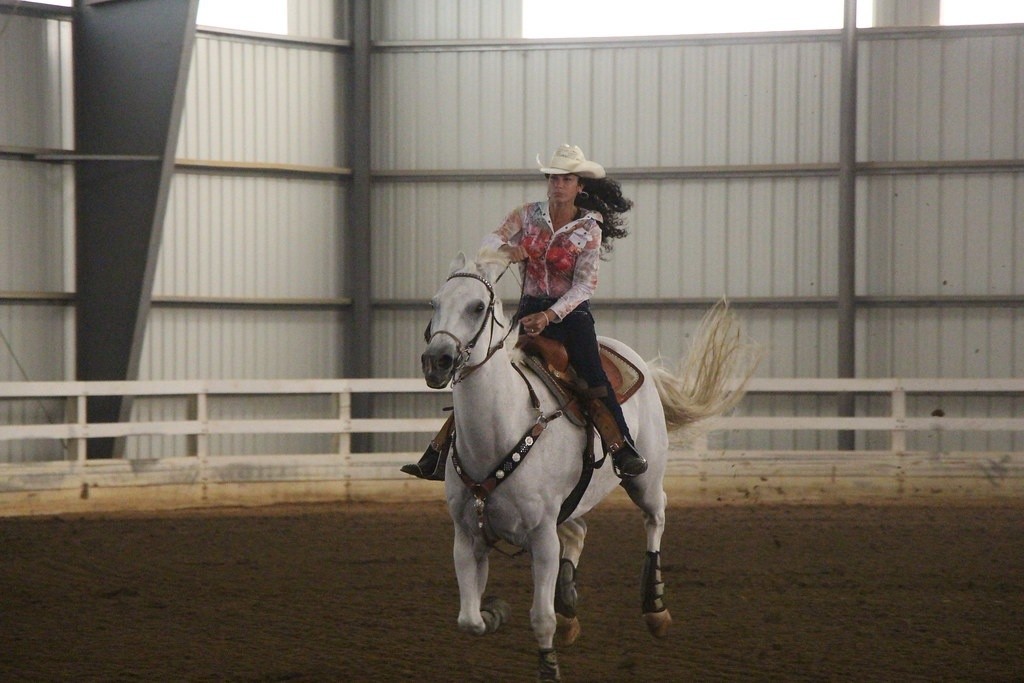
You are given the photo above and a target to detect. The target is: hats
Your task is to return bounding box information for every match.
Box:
[536,143,607,180]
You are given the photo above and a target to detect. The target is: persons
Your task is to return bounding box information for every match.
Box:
[400,144,648,481]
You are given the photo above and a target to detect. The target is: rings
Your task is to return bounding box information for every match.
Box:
[531,328,535,333]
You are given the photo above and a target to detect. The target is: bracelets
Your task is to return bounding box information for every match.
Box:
[540,312,549,326]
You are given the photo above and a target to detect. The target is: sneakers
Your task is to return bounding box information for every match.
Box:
[399,443,445,482]
[612,435,648,476]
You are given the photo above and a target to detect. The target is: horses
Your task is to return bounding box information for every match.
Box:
[420,250,764,682]
